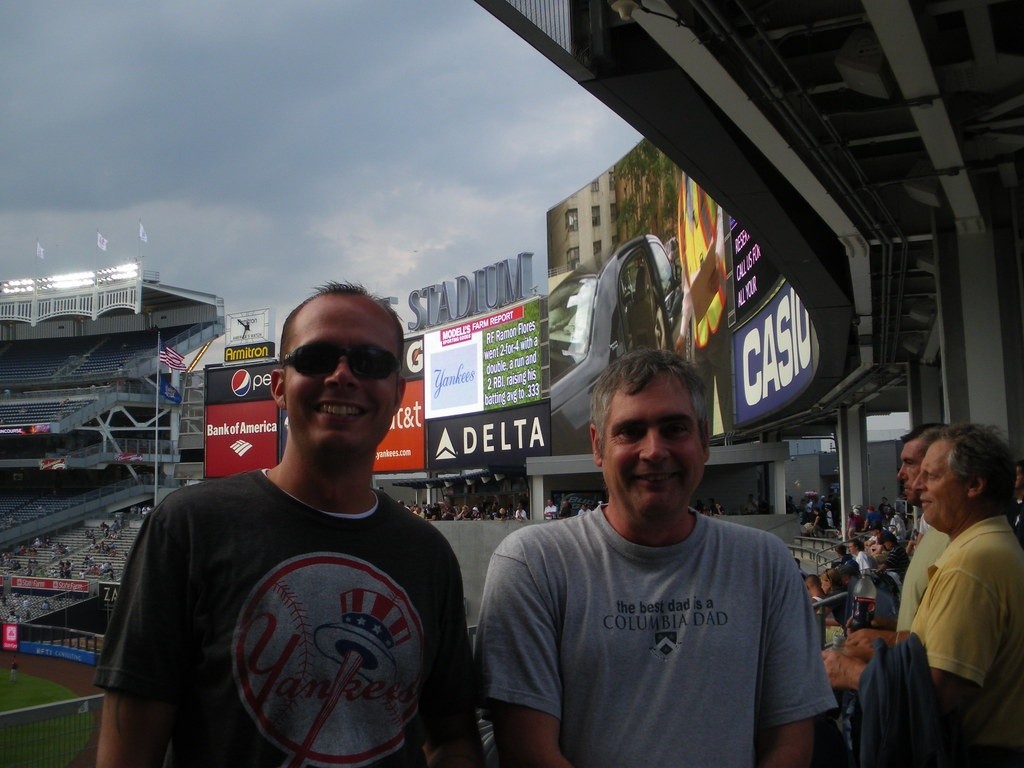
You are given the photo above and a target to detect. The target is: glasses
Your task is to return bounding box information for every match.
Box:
[281,341,399,381]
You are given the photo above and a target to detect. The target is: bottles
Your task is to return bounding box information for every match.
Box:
[850,569,878,630]
[823,633,851,719]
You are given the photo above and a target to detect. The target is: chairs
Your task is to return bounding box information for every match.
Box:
[0,320,213,621]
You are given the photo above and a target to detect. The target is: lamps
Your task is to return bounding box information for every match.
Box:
[888,364,902,374]
[444,481,455,488]
[481,477,492,484]
[915,241,935,274]
[465,479,476,486]
[902,158,949,209]
[902,335,927,354]
[835,28,896,101]
[425,484,434,489]
[910,300,931,324]
[494,473,506,481]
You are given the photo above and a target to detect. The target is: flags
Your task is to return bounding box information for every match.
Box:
[158,381,182,404]
[159,339,187,371]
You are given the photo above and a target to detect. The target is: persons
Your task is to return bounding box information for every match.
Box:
[0,587,52,624]
[95,283,475,768]
[475,350,841,768]
[11,660,17,681]
[792,423,1024,768]
[3,504,152,579]
[396,497,592,524]
